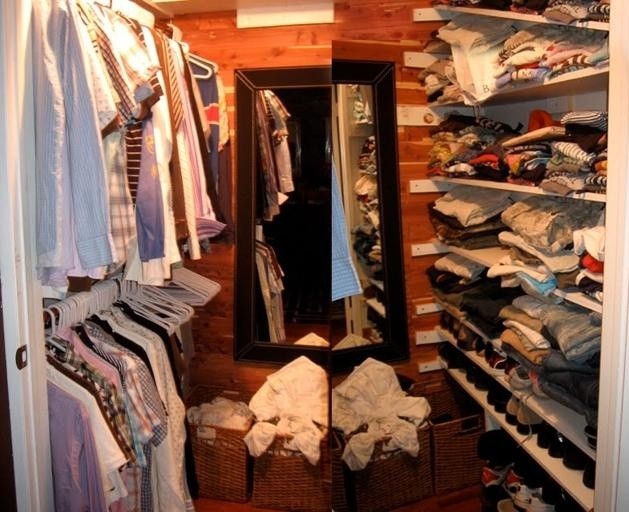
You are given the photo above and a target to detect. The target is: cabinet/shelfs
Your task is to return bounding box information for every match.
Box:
[436,0,628,512]
[339,86,388,343]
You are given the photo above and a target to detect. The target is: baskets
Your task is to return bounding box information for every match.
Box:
[188,379,483,512]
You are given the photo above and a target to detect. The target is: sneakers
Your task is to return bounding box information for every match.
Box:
[482,463,555,512]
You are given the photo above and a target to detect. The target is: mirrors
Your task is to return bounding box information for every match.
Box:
[333,57,409,366]
[233,67,336,364]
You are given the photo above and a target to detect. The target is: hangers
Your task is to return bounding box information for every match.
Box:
[42,265,222,405]
[28,0,222,78]
